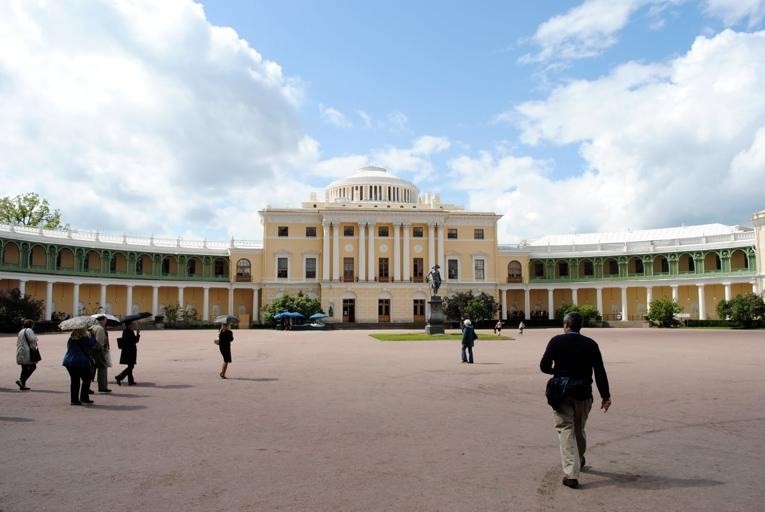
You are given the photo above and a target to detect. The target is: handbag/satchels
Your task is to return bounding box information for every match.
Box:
[30,346,41,362]
[88,350,97,368]
[103,350,114,368]
[545,375,572,411]
[473,331,478,341]
[116,337,125,349]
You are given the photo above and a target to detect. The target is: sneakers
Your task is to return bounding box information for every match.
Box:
[71,400,81,405]
[115,375,122,386]
[88,388,94,394]
[20,387,31,390]
[79,399,95,405]
[128,381,137,386]
[562,476,578,489]
[98,388,112,393]
[16,381,23,388]
[579,456,586,472]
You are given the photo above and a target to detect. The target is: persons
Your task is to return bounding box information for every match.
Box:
[494,318,507,335]
[15,319,40,390]
[51,307,141,406]
[212,322,234,378]
[459,318,479,364]
[540,311,613,489]
[428,264,442,295]
[520,321,527,335]
[273,316,320,330]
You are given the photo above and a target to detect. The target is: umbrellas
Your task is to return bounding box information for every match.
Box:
[273,312,327,319]
